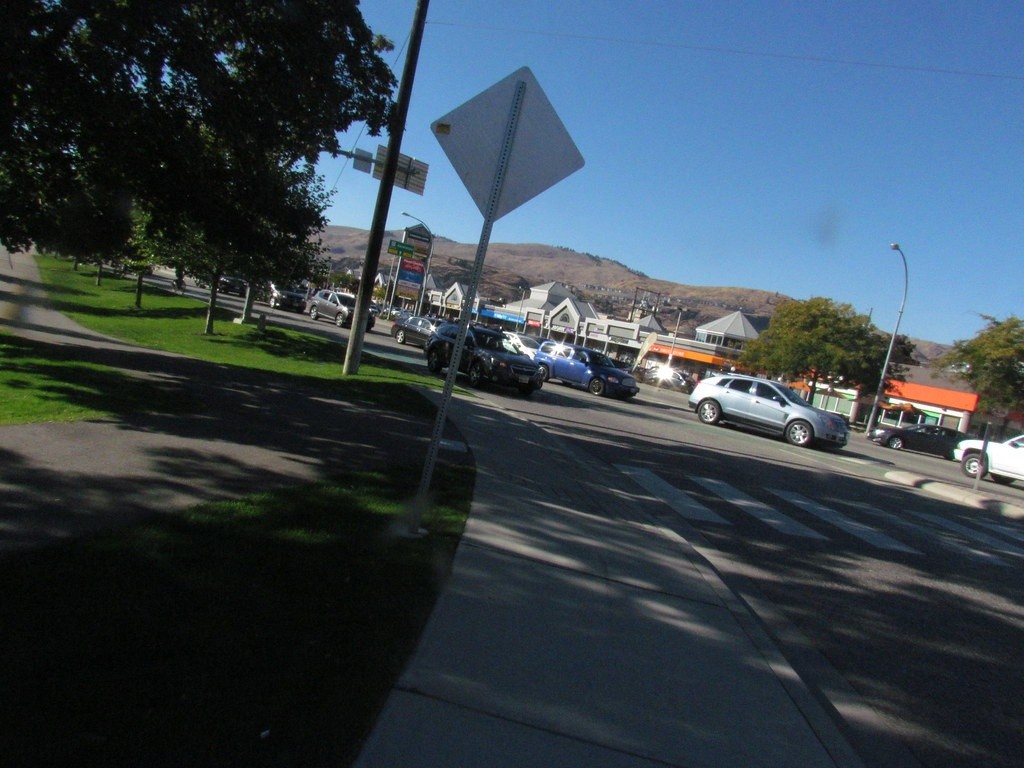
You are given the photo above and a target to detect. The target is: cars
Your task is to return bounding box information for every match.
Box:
[954,435,1024,483]
[867,423,970,461]
[487,329,540,360]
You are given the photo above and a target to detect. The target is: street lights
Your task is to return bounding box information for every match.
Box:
[515,286,525,328]
[666,307,684,368]
[864,244,910,436]
[402,212,435,316]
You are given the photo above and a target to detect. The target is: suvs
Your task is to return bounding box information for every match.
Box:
[424,317,541,397]
[534,341,642,399]
[687,373,850,449]
[192,267,447,351]
[644,365,694,392]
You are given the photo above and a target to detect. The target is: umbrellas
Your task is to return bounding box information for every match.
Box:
[784,381,930,438]
